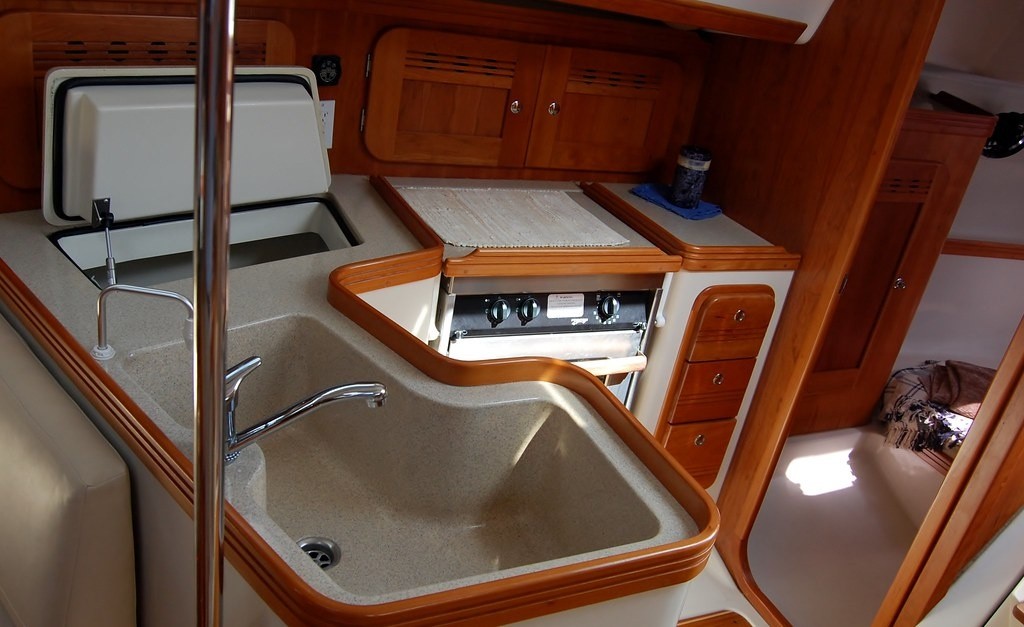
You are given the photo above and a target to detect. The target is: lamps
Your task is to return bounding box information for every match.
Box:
[911,77,1024,159]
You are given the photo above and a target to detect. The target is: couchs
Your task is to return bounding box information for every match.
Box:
[876,357,997,477]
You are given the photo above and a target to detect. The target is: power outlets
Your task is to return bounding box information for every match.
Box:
[320,99,336,149]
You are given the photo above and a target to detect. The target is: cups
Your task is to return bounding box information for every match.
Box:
[668,143,712,210]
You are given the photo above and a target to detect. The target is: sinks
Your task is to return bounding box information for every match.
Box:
[120,315,723,627]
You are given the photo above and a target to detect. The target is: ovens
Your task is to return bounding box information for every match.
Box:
[446,293,648,411]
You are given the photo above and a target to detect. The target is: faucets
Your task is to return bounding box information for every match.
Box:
[224,358,388,468]
[90,283,193,362]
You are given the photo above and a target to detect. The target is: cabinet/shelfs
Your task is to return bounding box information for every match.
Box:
[785,84,999,437]
[579,177,804,512]
